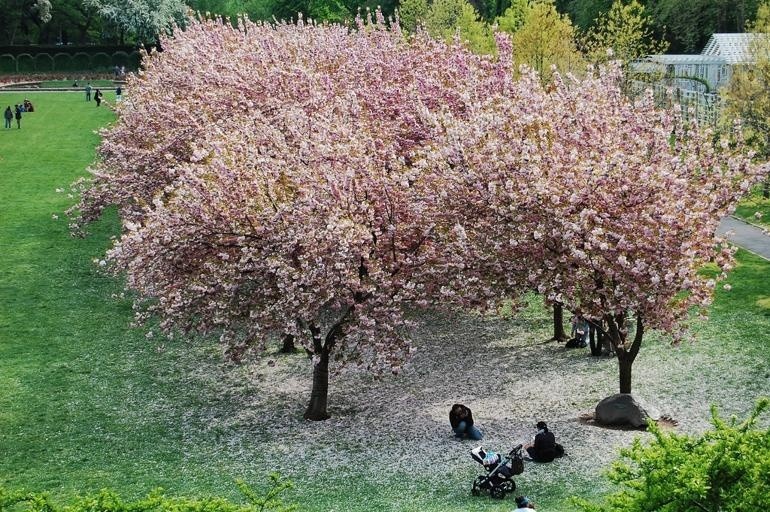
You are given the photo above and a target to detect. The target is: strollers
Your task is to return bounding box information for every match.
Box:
[469,442,526,501]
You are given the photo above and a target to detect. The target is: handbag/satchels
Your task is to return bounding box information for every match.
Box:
[566,338,587,349]
[512,450,524,475]
[553,442,564,458]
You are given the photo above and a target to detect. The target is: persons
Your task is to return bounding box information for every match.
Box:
[447,403,483,441]
[115,86,122,102]
[18,99,34,113]
[93,89,103,107]
[84,83,92,102]
[511,495,537,512]
[14,104,22,129]
[569,318,589,349]
[522,420,556,464]
[3,106,14,129]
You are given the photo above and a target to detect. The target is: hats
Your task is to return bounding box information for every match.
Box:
[516,496,530,505]
[536,421,547,429]
[575,307,586,315]
